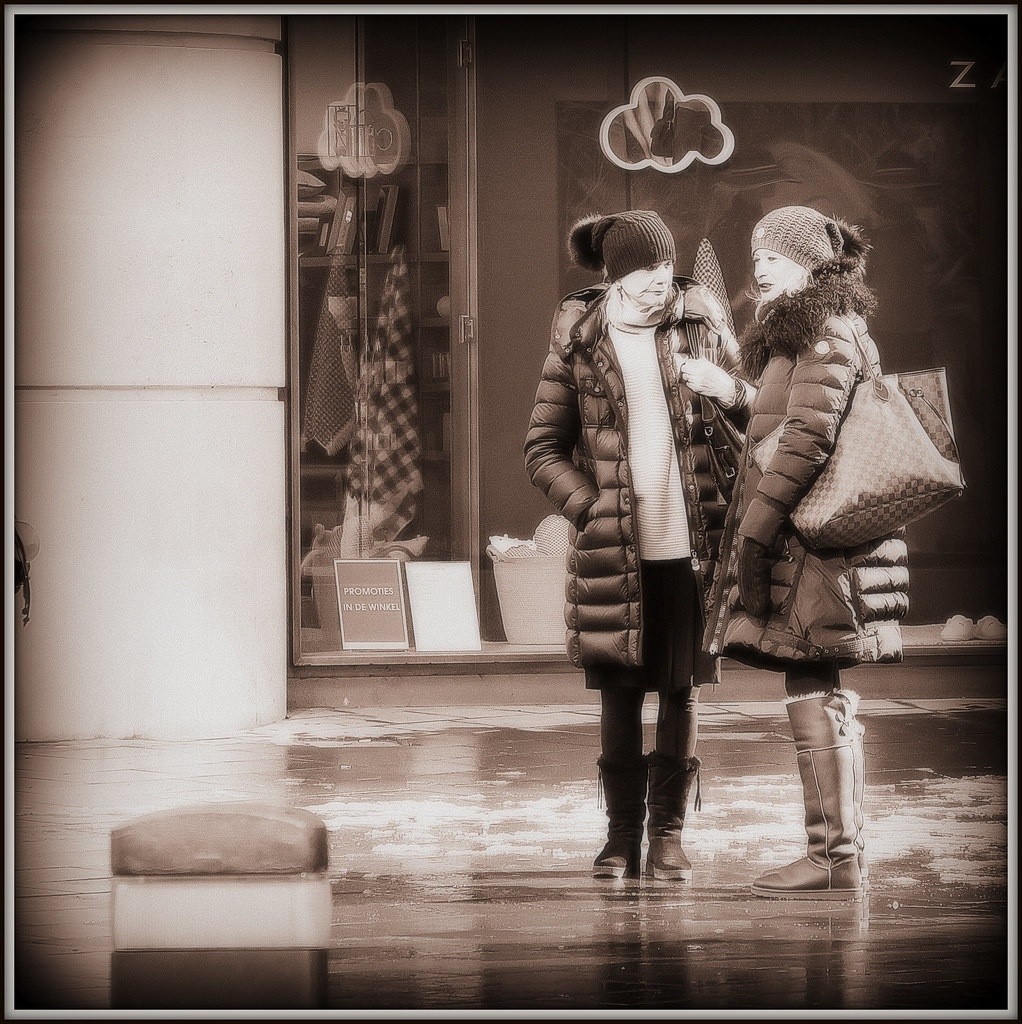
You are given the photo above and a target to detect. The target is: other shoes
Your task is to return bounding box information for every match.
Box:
[973,615,1006,639]
[942,616,974,641]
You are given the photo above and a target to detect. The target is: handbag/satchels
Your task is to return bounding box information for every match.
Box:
[684,318,747,504]
[748,314,965,548]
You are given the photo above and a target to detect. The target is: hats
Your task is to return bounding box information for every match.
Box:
[568,210,678,284]
[750,205,867,276]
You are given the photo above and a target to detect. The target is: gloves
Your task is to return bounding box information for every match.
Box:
[737,536,773,619]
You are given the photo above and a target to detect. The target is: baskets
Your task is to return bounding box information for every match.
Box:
[486,544,570,645]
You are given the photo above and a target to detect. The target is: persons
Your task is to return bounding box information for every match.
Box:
[701,204,909,900]
[523,210,757,880]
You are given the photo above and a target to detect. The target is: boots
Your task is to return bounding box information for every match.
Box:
[751,689,871,900]
[644,750,703,880]
[593,753,648,879]
